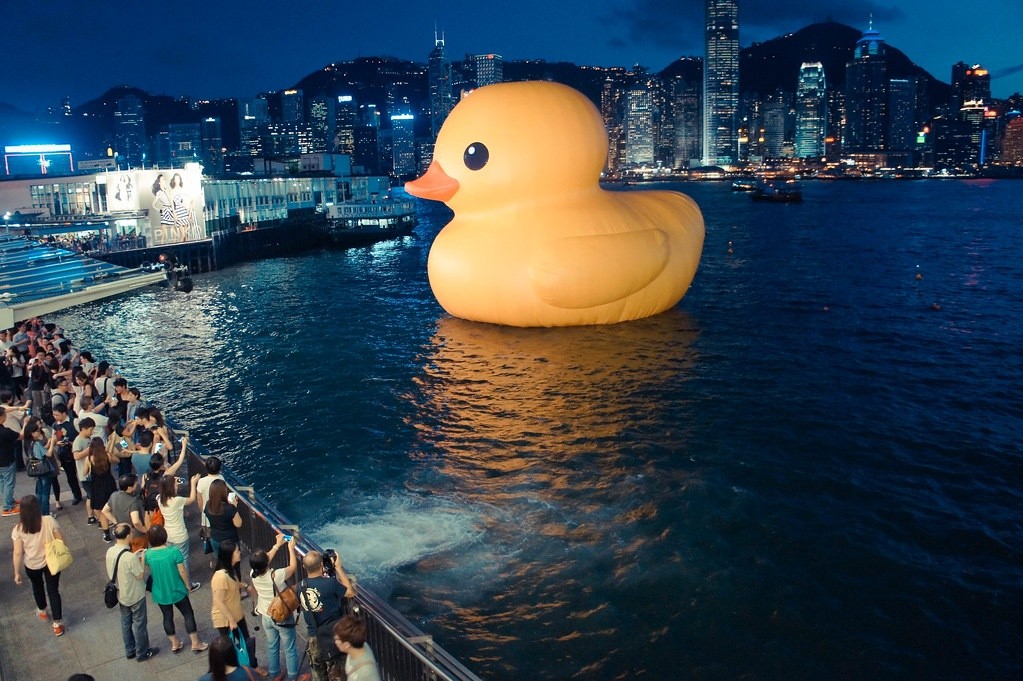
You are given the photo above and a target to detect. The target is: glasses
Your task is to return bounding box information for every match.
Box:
[333,637,342,641]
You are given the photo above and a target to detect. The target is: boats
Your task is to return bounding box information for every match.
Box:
[732,177,805,202]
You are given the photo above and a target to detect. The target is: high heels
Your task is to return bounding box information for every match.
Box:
[191,642,209,654]
[172,642,183,654]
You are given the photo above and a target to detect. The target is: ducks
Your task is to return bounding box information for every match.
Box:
[405,80,705,327]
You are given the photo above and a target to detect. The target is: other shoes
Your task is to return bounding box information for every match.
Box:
[2,507,21,516]
[138,647,160,662]
[13,498,21,506]
[255,663,311,681]
[188,582,200,592]
[53,625,64,636]
[127,653,136,660]
[16,465,27,472]
[14,400,22,406]
[37,608,48,623]
[47,497,116,543]
[239,589,248,600]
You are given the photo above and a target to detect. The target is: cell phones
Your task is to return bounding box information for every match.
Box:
[284,536,292,541]
[177,478,186,484]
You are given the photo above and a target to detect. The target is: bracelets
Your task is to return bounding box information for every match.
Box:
[273,546,278,550]
[103,401,107,404]
[17,406,18,411]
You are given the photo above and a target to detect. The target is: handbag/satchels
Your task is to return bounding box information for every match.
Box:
[203,539,214,555]
[104,428,136,457]
[228,626,259,669]
[151,507,164,526]
[44,516,73,576]
[94,395,109,417]
[26,441,52,477]
[266,570,300,623]
[103,580,119,608]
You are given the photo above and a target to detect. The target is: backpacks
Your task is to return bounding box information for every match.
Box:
[139,469,166,511]
[39,393,65,427]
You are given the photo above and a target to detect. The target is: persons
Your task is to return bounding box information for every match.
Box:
[41,234,127,251]
[0,317,381,681]
[152,173,200,244]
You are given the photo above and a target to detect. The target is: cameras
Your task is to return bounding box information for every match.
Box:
[62,439,72,449]
[321,549,336,568]
